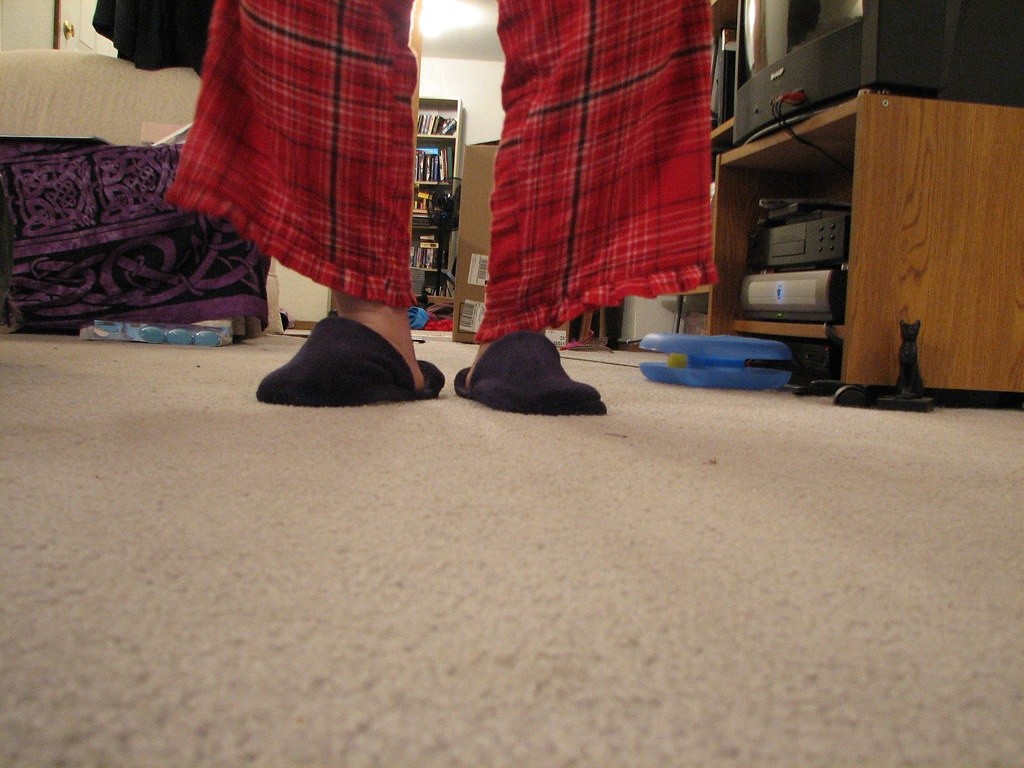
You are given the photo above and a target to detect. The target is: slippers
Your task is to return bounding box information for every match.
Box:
[256,317,447,407]
[453,331,609,416]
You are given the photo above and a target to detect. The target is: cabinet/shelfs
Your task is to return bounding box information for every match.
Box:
[707,94,1024,391]
[411,98,465,305]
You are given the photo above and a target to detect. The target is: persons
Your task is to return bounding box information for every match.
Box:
[168,0,718,416]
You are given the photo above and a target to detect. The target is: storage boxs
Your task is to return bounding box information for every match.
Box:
[453,141,569,347]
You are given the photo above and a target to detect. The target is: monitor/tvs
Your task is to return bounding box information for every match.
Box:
[732,0,943,147]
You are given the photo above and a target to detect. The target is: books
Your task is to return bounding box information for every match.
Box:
[411,234,446,270]
[415,147,453,182]
[413,190,441,226]
[426,287,445,296]
[418,114,457,134]
